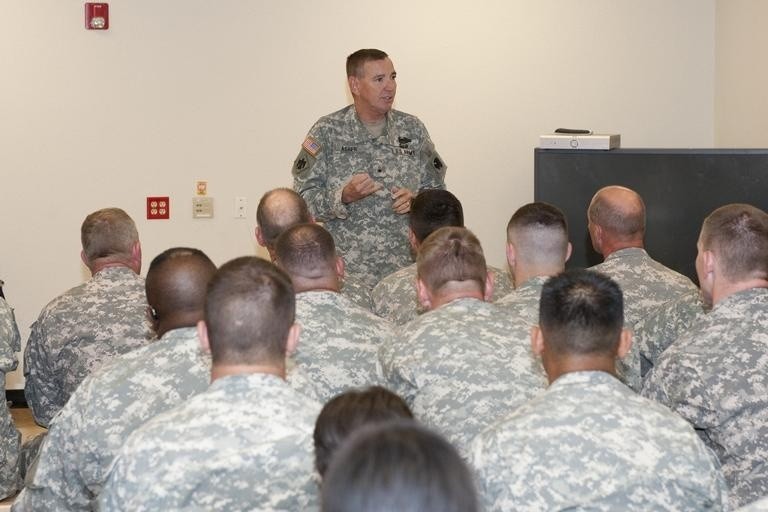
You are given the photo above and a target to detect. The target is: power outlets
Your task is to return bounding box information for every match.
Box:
[192,198,213,219]
[147,196,170,219]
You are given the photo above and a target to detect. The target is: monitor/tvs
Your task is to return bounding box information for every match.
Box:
[533,149,768,294]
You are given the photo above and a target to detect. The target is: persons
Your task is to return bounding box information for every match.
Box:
[291,48,447,309]
[0,185,768,512]
